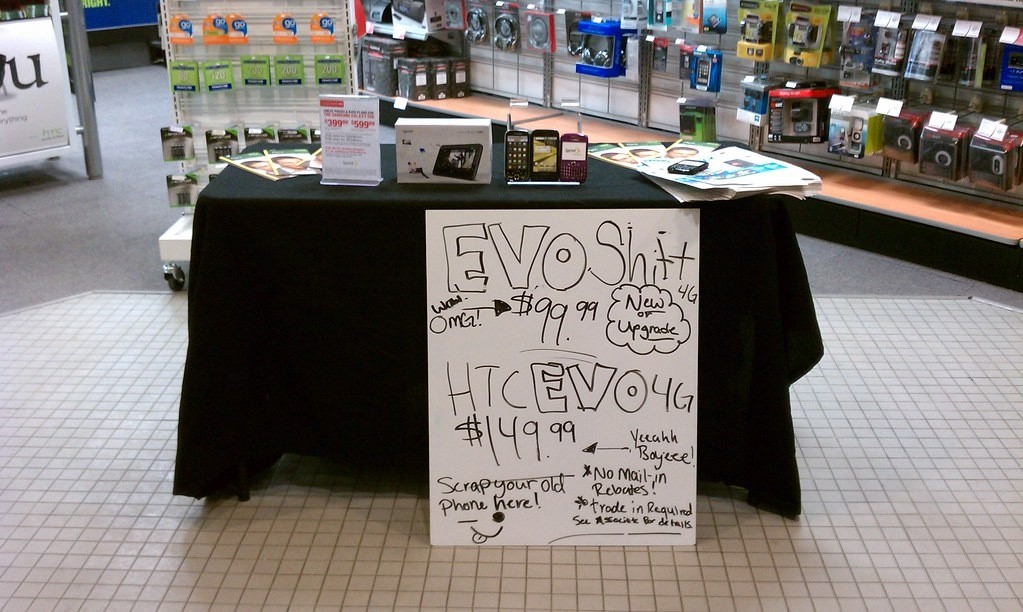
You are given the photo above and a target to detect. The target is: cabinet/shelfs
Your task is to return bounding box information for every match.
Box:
[0,0,104,182]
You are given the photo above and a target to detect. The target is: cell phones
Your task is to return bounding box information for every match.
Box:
[558,133,589,183]
[530,129,559,183]
[503,129,531,183]
[668,159,709,175]
[695,57,712,92]
[432,143,484,180]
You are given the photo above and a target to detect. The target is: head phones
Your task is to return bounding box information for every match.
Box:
[566,19,613,67]
[494,14,520,53]
[464,7,488,44]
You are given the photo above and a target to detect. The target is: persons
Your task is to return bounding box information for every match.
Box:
[630,148,661,159]
[272,155,322,175]
[241,159,276,176]
[601,153,638,165]
[310,152,323,168]
[664,146,698,158]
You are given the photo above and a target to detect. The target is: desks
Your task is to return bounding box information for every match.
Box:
[172,142,824,520]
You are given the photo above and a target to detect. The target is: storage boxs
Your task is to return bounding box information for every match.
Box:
[362,36,412,96]
[576,21,620,78]
[398,57,431,101]
[735,80,771,127]
[449,58,471,98]
[769,89,835,144]
[430,58,451,100]
[395,117,493,185]
[690,49,723,93]
